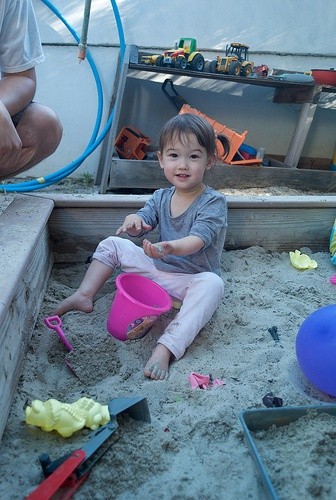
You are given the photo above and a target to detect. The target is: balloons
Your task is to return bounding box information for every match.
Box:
[295,304,336,396]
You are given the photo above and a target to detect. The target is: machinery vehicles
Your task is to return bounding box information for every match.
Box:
[157,38,206,72]
[211,41,253,77]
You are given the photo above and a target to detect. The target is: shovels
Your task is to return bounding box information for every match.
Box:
[44,315,97,381]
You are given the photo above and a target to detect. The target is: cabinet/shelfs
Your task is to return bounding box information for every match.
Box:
[91,43,335,196]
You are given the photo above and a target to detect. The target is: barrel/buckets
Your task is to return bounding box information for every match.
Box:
[106,273,173,342]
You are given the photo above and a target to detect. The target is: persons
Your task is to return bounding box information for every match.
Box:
[0,0,62,181]
[52,113,232,380]
